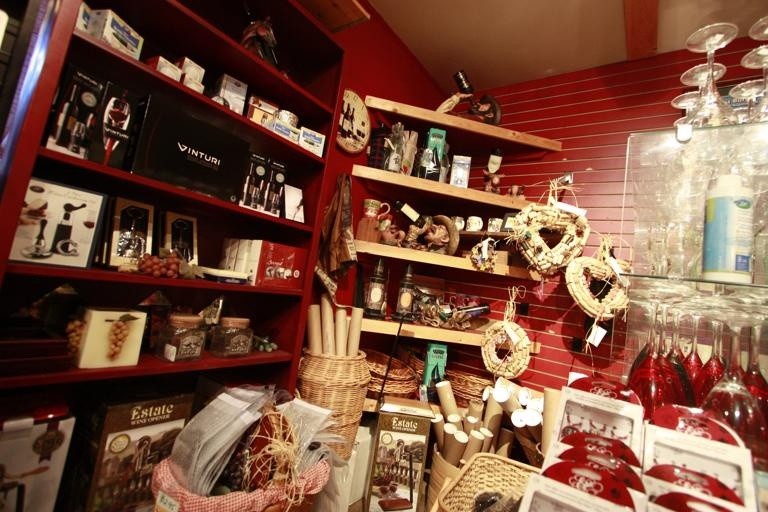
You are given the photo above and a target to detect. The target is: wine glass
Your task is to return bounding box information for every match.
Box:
[669,14,768,134]
[626,286,768,472]
[113,203,146,263]
[170,218,193,265]
[95,95,133,167]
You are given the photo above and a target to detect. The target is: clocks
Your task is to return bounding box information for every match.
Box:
[338,88,371,156]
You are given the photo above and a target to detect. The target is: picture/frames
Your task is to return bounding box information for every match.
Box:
[105,194,200,268]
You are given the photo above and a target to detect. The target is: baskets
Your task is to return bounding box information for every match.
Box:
[438,450,543,512]
[361,344,495,410]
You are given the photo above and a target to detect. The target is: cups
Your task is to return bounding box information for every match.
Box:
[356,118,367,142]
[450,214,466,231]
[67,120,87,153]
[486,217,504,232]
[360,197,391,220]
[465,215,484,232]
[250,188,260,210]
[270,193,280,215]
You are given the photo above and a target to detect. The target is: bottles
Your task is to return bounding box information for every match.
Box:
[242,162,256,206]
[384,122,405,173]
[389,260,420,324]
[392,198,435,233]
[54,82,80,149]
[359,255,392,322]
[209,316,256,359]
[699,171,759,285]
[263,169,275,212]
[49,201,91,256]
[158,312,211,362]
[334,98,355,139]
[412,286,492,331]
[453,68,479,109]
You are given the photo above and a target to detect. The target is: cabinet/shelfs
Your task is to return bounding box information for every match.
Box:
[337,93,561,427]
[0,0,347,493]
